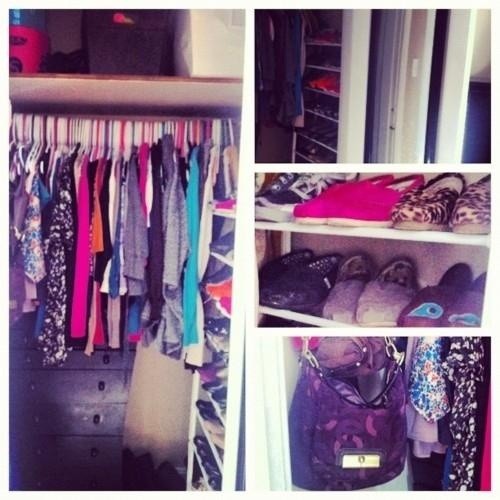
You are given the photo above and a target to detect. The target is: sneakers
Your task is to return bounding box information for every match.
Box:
[294,175,400,225]
[259,249,315,288]
[398,264,474,327]
[255,172,360,221]
[444,271,486,327]
[255,173,299,206]
[450,173,491,234]
[328,175,424,227]
[259,253,347,309]
[392,173,467,231]
[324,253,378,322]
[357,257,420,327]
[294,29,339,164]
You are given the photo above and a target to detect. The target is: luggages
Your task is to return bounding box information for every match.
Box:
[10,25,51,74]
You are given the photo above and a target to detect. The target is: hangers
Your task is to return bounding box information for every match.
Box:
[10,113,239,184]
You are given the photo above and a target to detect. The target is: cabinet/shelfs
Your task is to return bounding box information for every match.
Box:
[244,9,490,494]
[8,74,243,490]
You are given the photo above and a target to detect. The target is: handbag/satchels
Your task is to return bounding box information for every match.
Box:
[288,358,407,489]
